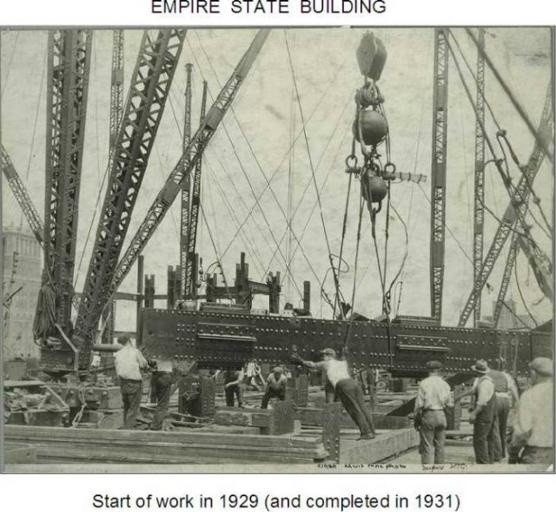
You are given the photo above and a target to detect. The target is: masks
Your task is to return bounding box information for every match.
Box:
[321,348,336,356]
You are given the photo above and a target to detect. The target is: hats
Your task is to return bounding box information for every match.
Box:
[471,359,490,374]
[528,357,553,377]
[426,360,442,370]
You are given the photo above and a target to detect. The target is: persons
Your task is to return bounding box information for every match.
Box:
[114,334,148,429]
[291,349,375,441]
[454,359,519,464]
[149,357,172,431]
[212,368,245,408]
[261,367,287,408]
[505,357,555,463]
[414,361,450,464]
